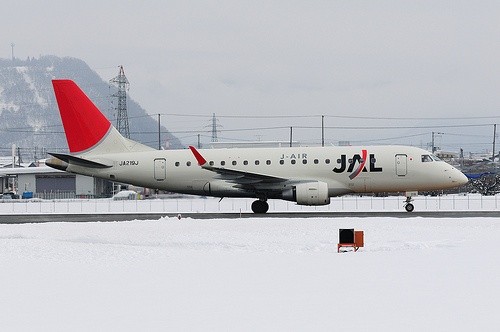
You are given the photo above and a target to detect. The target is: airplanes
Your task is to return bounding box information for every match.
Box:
[44,80,468,212]
